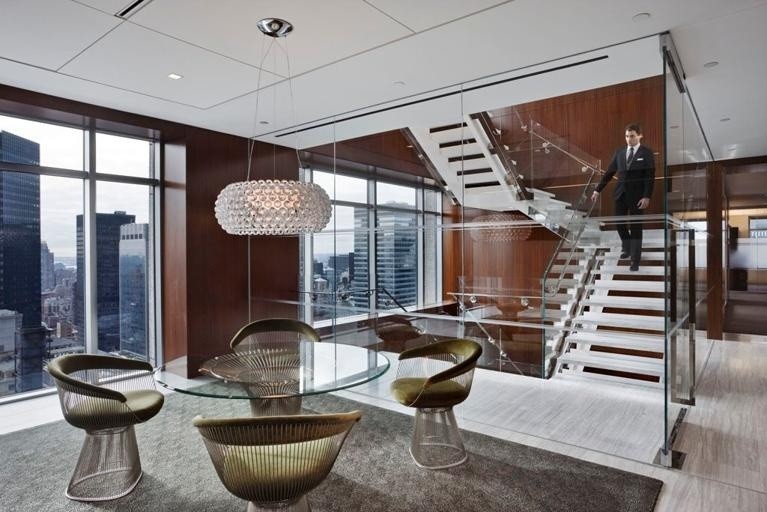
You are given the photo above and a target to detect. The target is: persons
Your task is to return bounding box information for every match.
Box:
[589,123,657,272]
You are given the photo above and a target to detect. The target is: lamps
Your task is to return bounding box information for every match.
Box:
[468,212,534,244]
[210,16,334,239]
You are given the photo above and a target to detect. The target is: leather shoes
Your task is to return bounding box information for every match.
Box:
[629,258,640,272]
[619,250,630,259]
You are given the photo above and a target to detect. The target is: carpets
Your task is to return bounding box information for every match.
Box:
[0,375,666,511]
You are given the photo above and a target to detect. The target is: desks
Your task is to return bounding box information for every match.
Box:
[152,338,392,417]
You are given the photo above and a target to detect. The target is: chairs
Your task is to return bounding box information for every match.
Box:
[41,351,169,502]
[228,315,322,417]
[186,408,369,511]
[388,337,485,470]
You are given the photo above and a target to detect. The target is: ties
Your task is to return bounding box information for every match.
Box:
[627,147,635,165]
